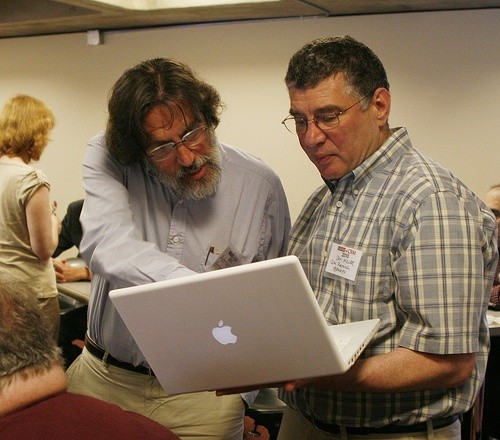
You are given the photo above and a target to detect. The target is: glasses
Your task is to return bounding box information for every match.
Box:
[141,111,210,162]
[281,86,385,135]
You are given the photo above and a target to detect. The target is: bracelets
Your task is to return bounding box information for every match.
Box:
[85,267,90,279]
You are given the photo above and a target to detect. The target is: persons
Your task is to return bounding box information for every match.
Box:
[0,94,60,347]
[64,57,292,440]
[0,268,182,440]
[276,34,500,440]
[51,199,92,282]
[480,185,500,310]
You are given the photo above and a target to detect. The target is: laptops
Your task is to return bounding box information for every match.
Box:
[108,255,381,394]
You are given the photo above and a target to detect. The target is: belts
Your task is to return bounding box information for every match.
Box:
[302,412,459,436]
[85,335,157,380]
